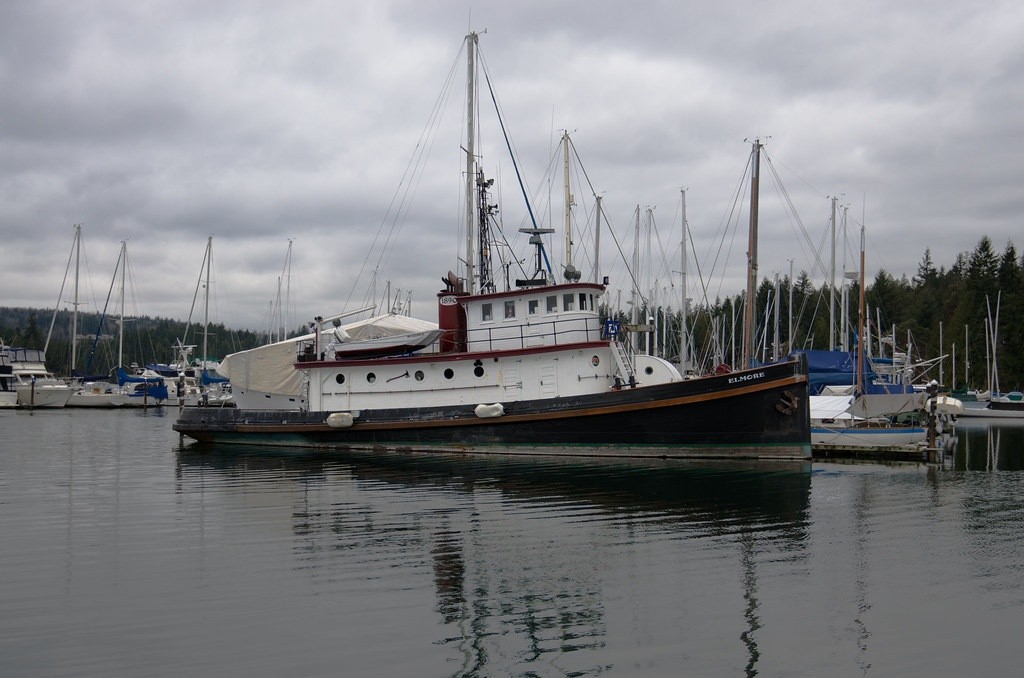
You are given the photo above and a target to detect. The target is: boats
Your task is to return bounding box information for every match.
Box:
[0,336,21,408]
[11,348,84,408]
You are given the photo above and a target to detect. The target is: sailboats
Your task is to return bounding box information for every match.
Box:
[43,222,132,407]
[580,194,1023,434]
[172,32,813,476]
[85,240,168,407]
[136,236,292,407]
